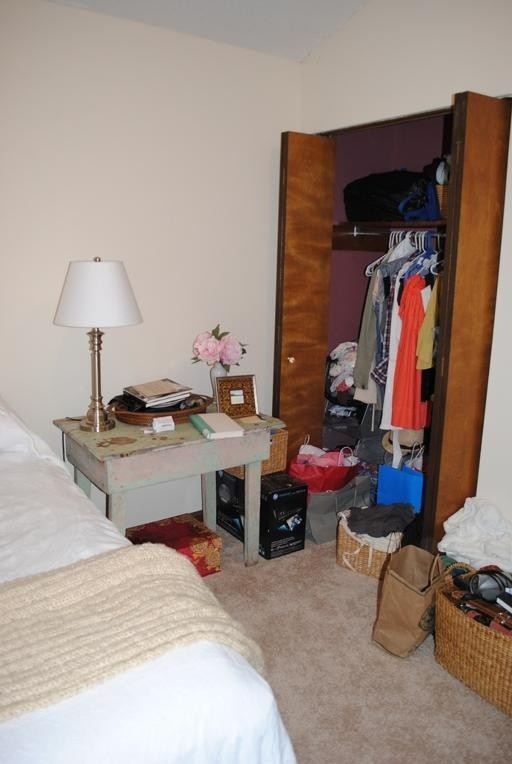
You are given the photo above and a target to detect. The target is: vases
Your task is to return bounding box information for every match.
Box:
[209,362,228,404]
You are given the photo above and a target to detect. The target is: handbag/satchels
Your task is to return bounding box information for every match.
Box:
[372,533,446,659]
[376,441,425,519]
[288,433,360,492]
[305,476,370,545]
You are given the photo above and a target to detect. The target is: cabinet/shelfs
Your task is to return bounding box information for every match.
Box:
[269,90,511,559]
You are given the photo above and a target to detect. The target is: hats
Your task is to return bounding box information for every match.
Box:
[382,429,424,455]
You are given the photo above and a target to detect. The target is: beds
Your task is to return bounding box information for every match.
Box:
[1,405,302,764]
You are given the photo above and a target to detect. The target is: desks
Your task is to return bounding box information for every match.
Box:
[51,413,275,568]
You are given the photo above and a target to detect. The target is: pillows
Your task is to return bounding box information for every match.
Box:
[1,402,54,454]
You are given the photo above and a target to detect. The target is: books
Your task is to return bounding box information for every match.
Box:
[496,592,512,613]
[125,378,193,404]
[189,412,244,439]
[123,391,191,409]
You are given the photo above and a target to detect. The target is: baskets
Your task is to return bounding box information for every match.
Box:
[106,394,213,427]
[223,429,289,479]
[434,563,512,717]
[336,524,391,581]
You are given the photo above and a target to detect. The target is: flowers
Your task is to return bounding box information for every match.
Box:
[190,323,253,373]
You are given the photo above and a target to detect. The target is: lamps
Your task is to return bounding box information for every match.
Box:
[51,257,145,433]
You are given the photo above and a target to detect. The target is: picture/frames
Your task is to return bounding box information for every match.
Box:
[213,374,257,420]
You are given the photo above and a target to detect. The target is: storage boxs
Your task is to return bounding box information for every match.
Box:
[125,514,221,580]
[215,469,309,561]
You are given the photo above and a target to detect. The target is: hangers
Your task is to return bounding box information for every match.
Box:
[416,232,443,276]
[399,230,405,242]
[366,231,393,274]
[409,232,429,270]
[418,232,422,253]
[430,260,444,276]
[410,232,427,264]
[414,232,419,249]
[405,232,438,278]
[364,231,396,278]
[381,231,401,264]
